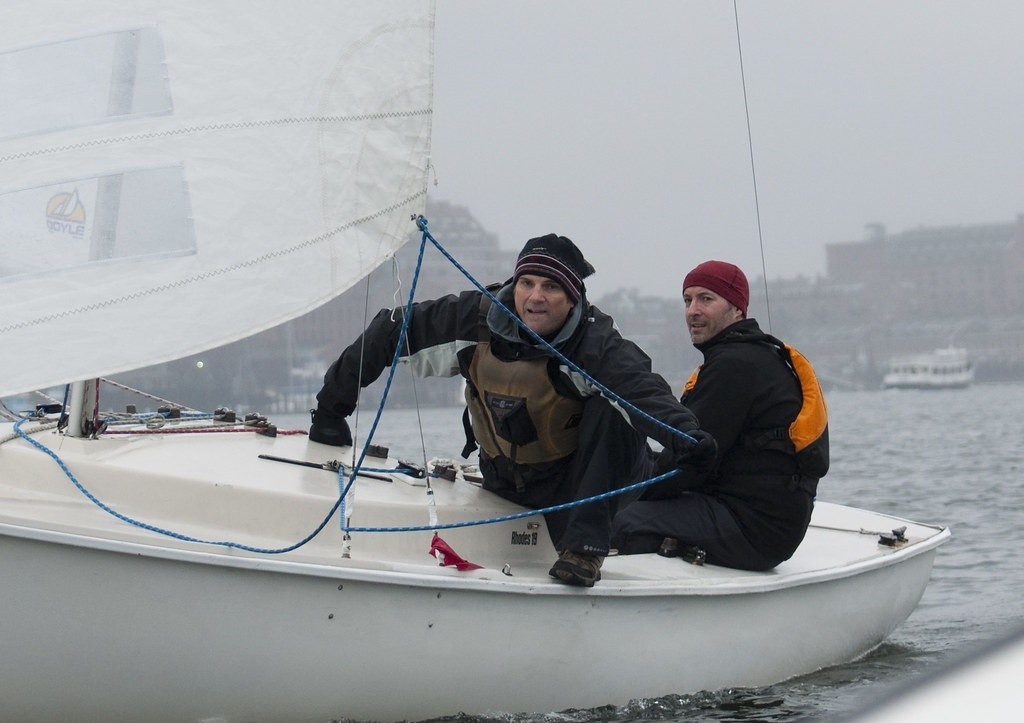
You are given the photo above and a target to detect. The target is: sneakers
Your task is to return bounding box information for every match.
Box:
[549,549,605,588]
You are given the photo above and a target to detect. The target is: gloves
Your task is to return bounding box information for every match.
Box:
[675,430,719,476]
[308,402,352,447]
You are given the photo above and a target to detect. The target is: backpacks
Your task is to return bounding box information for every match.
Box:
[750,332,829,477]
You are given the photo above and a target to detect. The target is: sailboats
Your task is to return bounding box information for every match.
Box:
[2,1,953,723]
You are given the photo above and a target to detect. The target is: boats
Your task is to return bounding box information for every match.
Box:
[882,343,977,389]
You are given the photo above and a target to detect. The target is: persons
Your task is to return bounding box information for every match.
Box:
[609,259,830,572]
[308,234,720,587]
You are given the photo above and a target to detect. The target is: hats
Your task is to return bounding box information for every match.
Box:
[683,259,750,319]
[514,232,586,306]
